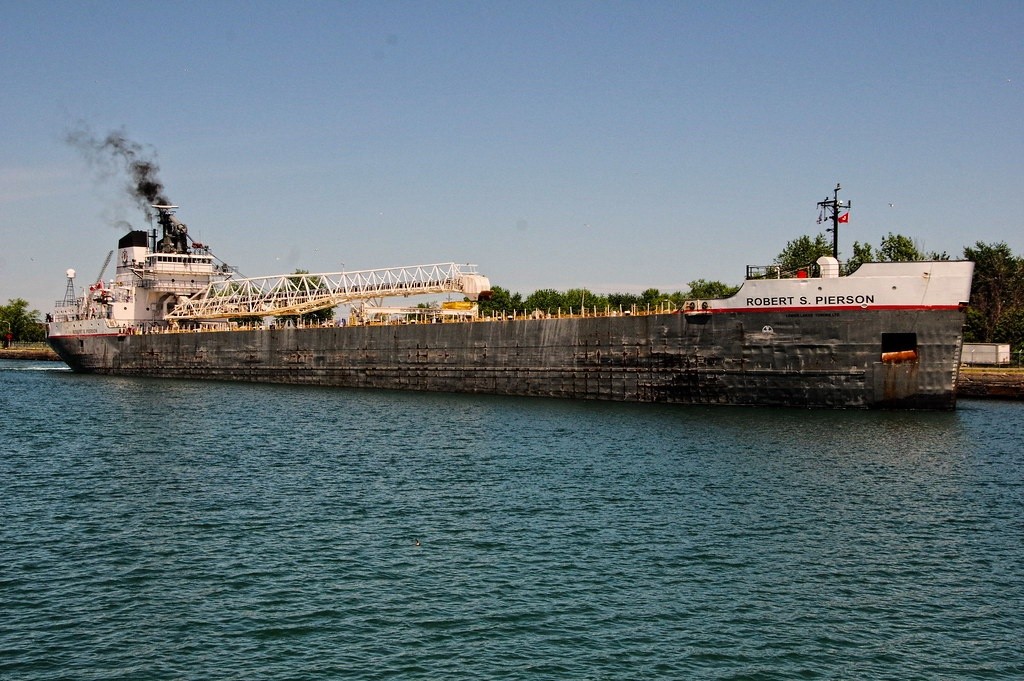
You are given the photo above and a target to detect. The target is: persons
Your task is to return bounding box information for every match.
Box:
[123,323,135,335]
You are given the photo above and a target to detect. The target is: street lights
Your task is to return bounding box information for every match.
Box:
[3,321,10,347]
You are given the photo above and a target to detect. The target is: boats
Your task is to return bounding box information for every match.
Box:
[45,180,975,411]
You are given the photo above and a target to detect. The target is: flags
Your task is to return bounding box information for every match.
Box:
[839,213,848,223]
